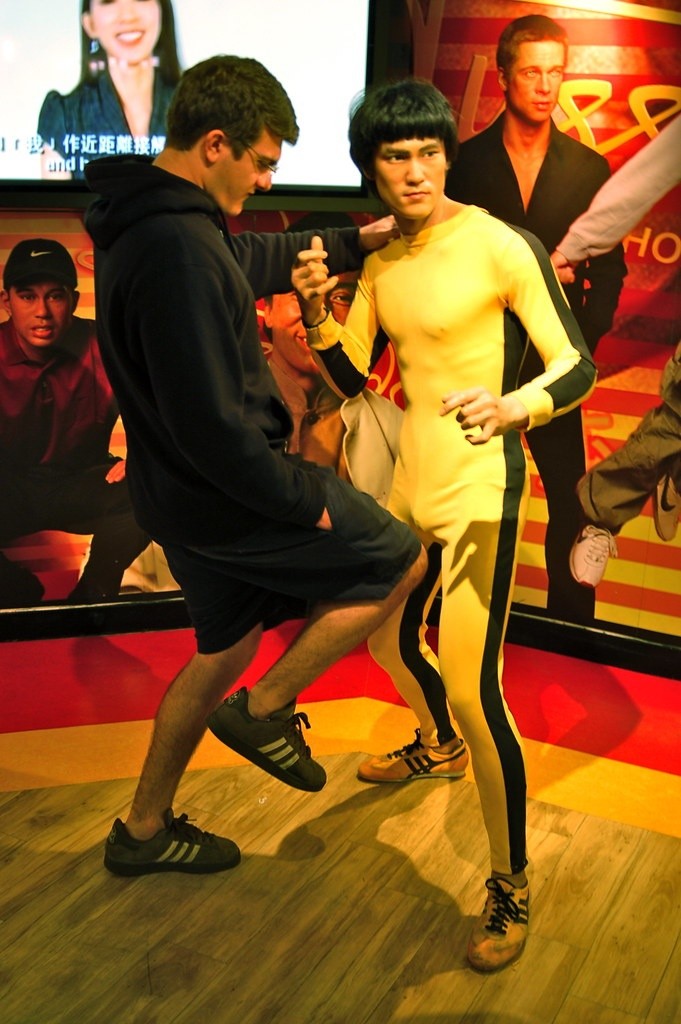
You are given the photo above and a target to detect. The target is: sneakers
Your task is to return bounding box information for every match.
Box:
[569,522,617,587]
[467,878,530,972]
[205,686,327,793]
[359,728,469,782]
[652,474,681,541]
[104,807,241,876]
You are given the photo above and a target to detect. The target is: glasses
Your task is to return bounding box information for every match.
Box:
[225,133,280,179]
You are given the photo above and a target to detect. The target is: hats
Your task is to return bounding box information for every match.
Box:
[3,239,78,290]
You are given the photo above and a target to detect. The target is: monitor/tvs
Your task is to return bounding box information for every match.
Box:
[0,0,388,213]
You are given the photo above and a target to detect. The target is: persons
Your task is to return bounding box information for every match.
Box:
[549,116,680,588]
[77,56,429,875]
[38,0,182,180]
[0,238,152,608]
[444,13,627,621]
[264,213,404,526]
[292,81,598,971]
